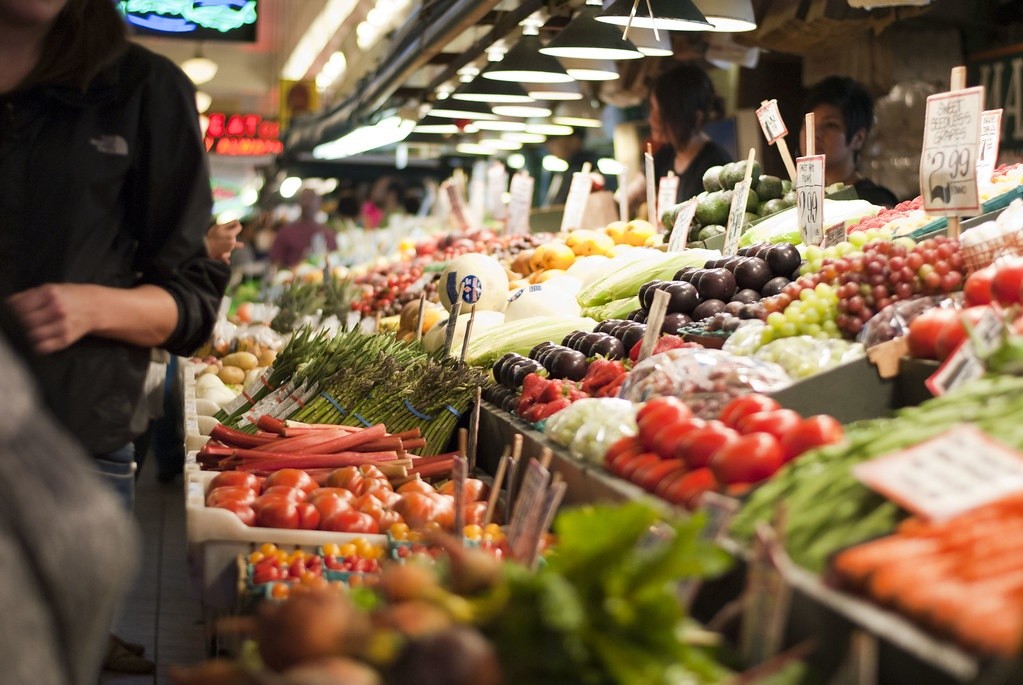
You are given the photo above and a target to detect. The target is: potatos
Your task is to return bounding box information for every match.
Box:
[194,351,276,384]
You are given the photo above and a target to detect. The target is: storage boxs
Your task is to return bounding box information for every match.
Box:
[176,183,896,591]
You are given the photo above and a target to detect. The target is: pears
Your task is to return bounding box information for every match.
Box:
[512,217,664,285]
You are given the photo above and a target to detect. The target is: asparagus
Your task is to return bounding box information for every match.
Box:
[206,323,489,456]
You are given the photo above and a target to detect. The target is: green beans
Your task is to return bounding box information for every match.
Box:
[730,330,1023,572]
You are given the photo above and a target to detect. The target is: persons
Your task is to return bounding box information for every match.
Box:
[796,75,900,210]
[95,217,246,677]
[612,61,738,208]
[269,175,409,273]
[545,122,603,206]
[0,0,232,523]
[0,340,145,684]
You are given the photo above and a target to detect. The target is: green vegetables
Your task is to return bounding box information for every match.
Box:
[268,259,363,333]
[461,490,833,685]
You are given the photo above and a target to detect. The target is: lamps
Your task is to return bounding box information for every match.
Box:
[491,97,552,118]
[554,83,603,127]
[452,61,534,103]
[481,35,574,83]
[538,10,645,60]
[594,0,715,31]
[558,57,622,80]
[529,82,579,100]
[412,115,459,133]
[620,25,674,57]
[427,89,497,120]
[692,0,757,32]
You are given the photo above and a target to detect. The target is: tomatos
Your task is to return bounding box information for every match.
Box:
[904,252,1023,367]
[204,463,490,536]
[248,521,556,599]
[602,392,840,507]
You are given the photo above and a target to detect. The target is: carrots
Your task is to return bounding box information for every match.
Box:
[830,486,1023,667]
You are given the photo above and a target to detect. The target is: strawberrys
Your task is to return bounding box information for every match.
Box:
[352,265,424,312]
[514,333,706,423]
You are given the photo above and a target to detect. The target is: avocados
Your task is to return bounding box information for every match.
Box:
[655,158,797,245]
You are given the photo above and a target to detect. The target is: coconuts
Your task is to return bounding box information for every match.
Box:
[421,253,581,354]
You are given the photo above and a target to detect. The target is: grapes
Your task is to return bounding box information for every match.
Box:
[751,225,964,348]
